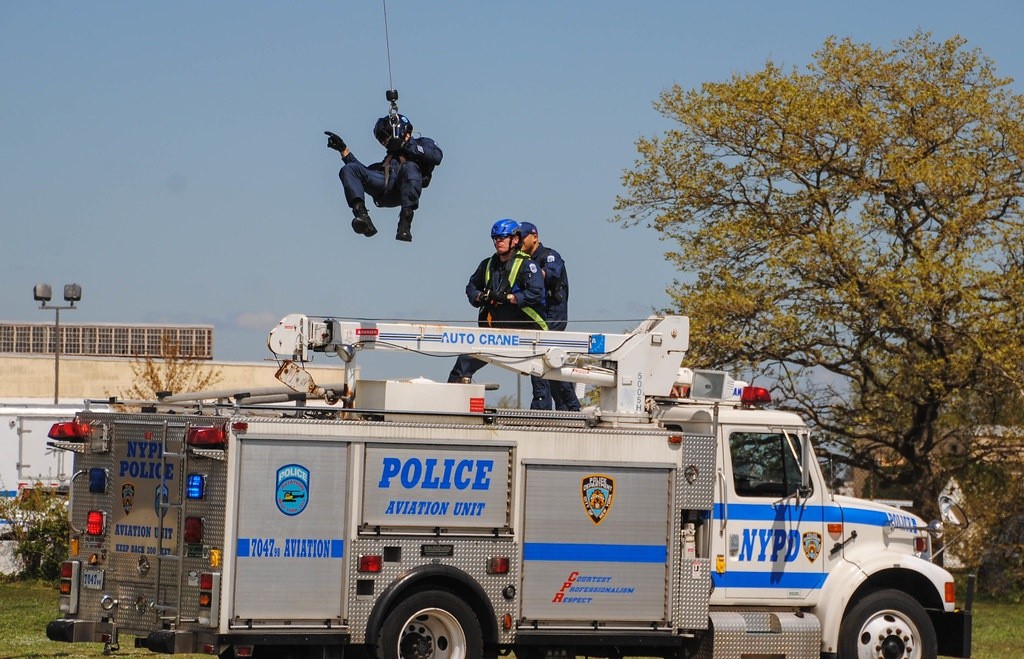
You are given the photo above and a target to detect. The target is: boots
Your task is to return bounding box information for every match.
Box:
[351,201,377,237]
[396,208,414,241]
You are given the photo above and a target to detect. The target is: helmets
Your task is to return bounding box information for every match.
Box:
[491,219,522,236]
[374,114,413,149]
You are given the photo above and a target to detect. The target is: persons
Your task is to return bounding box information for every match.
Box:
[324,113,443,242]
[448,219,582,412]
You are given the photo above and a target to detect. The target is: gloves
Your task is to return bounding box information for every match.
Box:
[324,132,346,152]
[386,137,404,153]
[480,287,511,310]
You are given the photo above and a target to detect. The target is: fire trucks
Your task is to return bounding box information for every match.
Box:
[42,315,981,659]
[0,398,121,501]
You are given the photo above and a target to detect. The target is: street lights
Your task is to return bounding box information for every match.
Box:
[32,282,84,405]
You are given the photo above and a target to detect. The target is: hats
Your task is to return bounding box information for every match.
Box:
[519,222,538,238]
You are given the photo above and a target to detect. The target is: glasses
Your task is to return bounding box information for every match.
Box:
[494,236,507,240]
